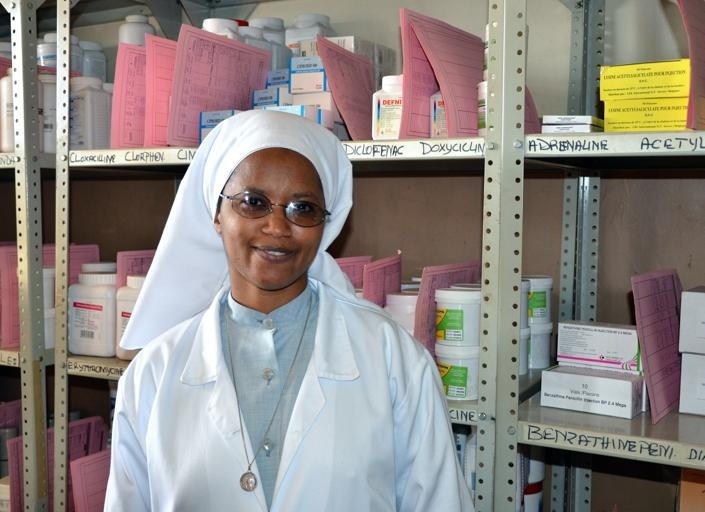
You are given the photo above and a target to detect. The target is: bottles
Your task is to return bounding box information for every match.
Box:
[201,10,340,69]
[117,13,156,48]
[0,427,19,478]
[369,72,446,141]
[522,445,547,512]
[381,275,422,338]
[0,33,115,155]
[41,260,147,362]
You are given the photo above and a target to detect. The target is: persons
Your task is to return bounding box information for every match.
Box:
[101,109,477,512]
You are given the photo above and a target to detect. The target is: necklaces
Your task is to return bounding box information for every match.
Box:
[224,283,313,491]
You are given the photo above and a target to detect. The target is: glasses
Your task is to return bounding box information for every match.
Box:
[219,192,330,227]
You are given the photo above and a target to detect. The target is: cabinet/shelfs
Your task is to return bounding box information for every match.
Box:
[490,0,705,512]
[0,0,182,512]
[53,0,587,512]
